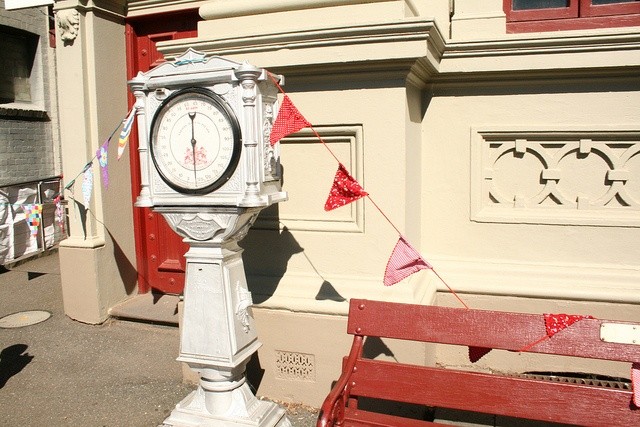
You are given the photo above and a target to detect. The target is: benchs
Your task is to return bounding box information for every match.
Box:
[315,298,640,427]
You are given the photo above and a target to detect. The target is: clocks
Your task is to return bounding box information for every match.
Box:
[147,86,244,197]
